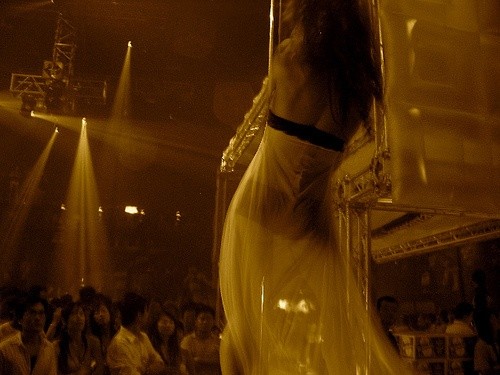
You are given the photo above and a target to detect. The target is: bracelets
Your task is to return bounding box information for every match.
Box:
[49,323,56,326]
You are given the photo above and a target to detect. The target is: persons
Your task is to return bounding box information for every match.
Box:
[219,0,385,375]
[0,284,500,375]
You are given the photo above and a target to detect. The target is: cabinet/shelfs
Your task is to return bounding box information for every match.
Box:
[392,334,478,375]
